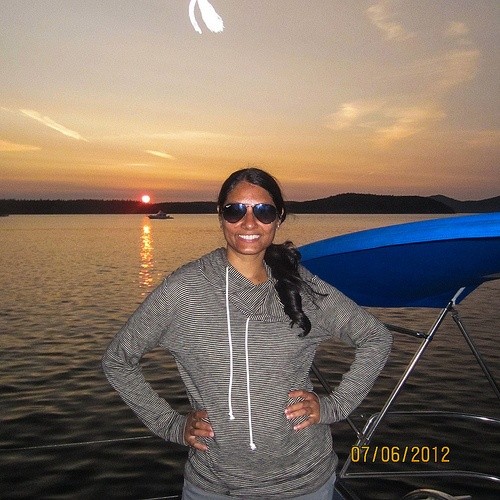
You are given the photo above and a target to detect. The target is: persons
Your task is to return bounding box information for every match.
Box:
[101,167,397,500]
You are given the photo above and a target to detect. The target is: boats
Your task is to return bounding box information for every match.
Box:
[148,210,174,219]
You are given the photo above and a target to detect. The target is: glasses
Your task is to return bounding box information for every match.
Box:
[219,203,281,225]
